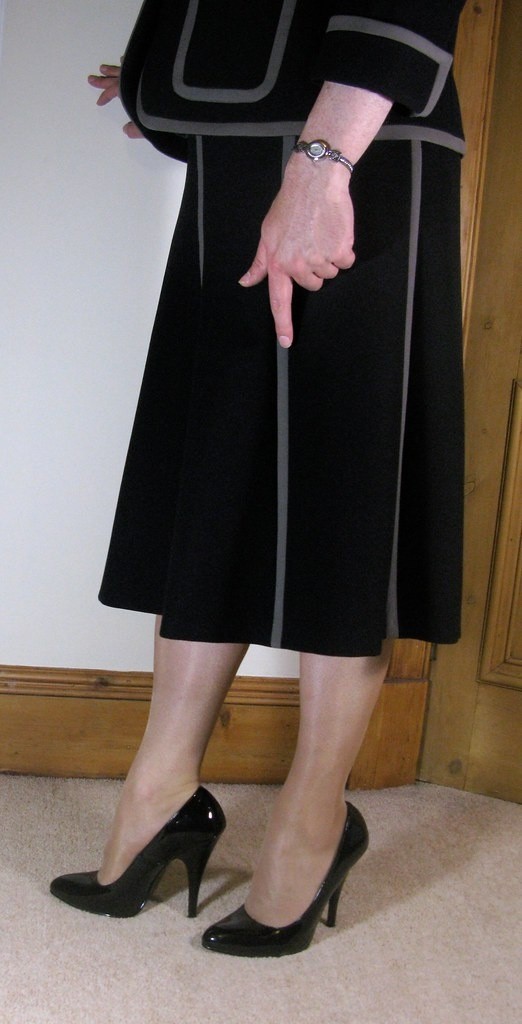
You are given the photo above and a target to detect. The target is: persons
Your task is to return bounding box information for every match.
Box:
[48,2,470,960]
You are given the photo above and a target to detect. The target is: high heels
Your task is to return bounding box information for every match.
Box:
[49,788,228,918]
[199,799,369,956]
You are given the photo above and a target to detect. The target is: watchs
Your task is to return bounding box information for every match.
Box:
[296,139,353,172]
